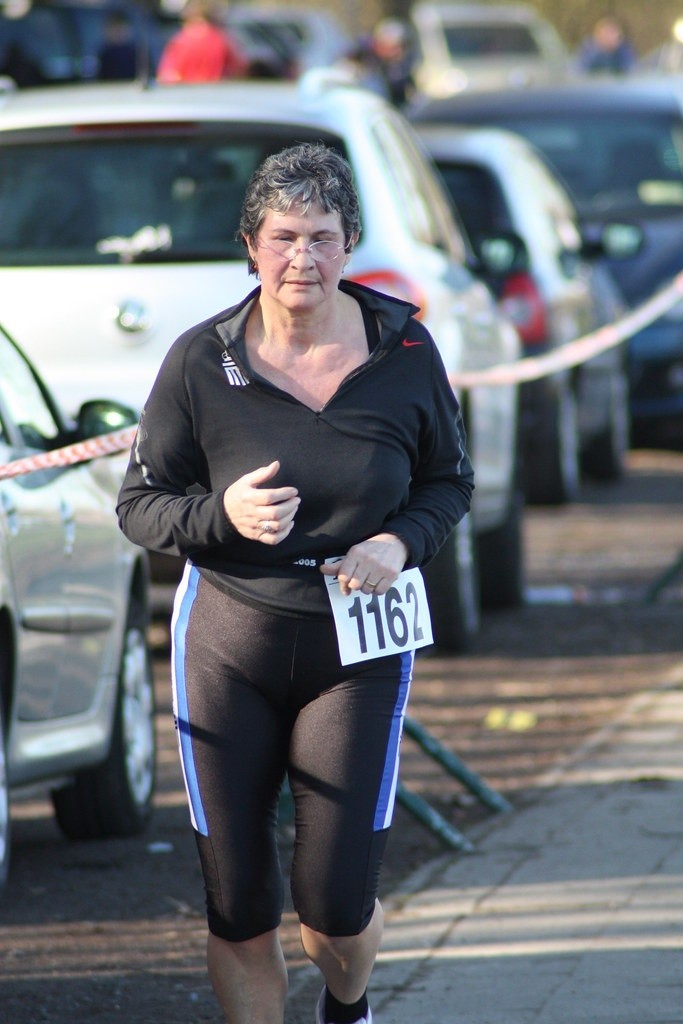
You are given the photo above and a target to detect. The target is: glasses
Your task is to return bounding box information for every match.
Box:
[261,231,354,262]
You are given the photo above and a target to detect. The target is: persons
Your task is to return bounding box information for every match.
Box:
[115,145,476,1024]
[156,0,249,82]
[96,16,141,80]
[344,23,413,104]
[579,20,637,75]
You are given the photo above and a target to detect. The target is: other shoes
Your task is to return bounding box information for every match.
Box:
[314,983,372,1024]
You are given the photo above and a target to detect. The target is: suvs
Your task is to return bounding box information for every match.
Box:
[0,64,534,662]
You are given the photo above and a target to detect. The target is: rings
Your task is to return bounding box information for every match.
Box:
[262,521,273,534]
[365,580,376,588]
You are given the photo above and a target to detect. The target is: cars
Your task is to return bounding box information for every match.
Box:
[0,332,157,852]
[107,2,683,505]
